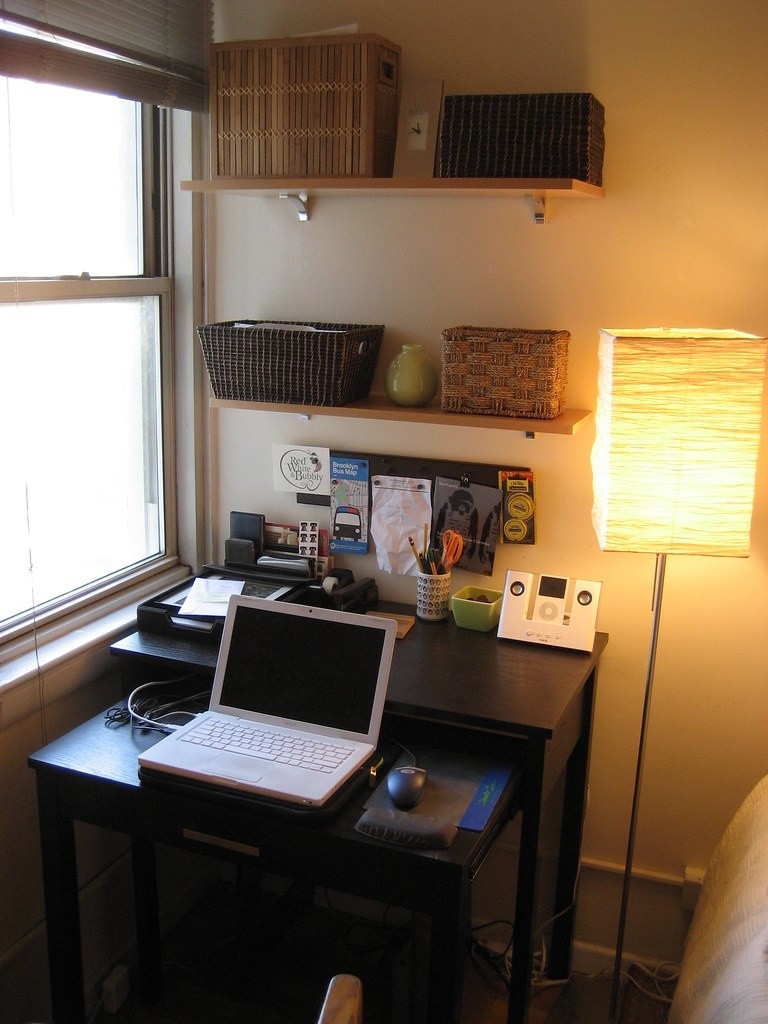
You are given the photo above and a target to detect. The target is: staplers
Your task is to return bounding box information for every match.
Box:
[333,577,378,614]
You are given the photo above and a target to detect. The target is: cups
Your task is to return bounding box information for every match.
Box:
[416,571,452,622]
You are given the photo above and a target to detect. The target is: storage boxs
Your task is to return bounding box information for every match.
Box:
[102,963,131,1013]
[451,586,503,631]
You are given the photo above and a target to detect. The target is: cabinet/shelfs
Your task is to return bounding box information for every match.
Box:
[177,178,608,434]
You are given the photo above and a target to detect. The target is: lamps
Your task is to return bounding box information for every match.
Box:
[585,326,767,1024]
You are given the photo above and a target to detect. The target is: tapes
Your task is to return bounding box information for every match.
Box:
[321,577,339,597]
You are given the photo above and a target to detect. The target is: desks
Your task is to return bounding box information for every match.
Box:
[28,596,609,1024]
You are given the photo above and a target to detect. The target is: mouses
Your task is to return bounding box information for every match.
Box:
[387,767,428,812]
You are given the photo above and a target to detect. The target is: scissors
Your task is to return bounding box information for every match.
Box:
[438,529,464,575]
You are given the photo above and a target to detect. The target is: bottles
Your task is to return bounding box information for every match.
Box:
[385,343,438,408]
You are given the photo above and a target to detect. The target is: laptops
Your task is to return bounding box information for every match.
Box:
[136,594,398,809]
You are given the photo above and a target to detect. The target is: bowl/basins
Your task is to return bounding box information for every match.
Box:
[451,586,504,632]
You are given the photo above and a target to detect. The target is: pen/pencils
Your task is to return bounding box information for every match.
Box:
[408,522,441,575]
